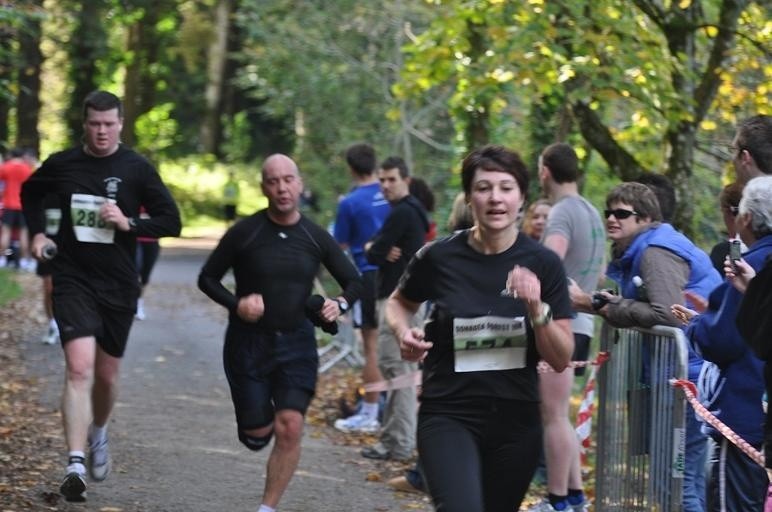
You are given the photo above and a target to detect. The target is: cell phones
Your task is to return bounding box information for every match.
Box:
[730,240,741,273]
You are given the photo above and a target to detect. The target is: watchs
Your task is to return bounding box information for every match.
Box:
[128,217,136,234]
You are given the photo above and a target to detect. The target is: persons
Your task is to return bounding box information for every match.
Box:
[197,153,363,512]
[19,90,182,502]
[330,115,771,512]
[221,172,240,230]
[0,149,161,343]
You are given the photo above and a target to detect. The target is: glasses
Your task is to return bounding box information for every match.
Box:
[605,210,637,219]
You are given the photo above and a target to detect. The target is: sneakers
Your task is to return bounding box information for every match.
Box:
[528,497,588,512]
[42,327,59,343]
[335,416,425,492]
[134,304,144,320]
[60,422,108,502]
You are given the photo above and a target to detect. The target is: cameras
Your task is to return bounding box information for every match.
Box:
[592,289,614,309]
